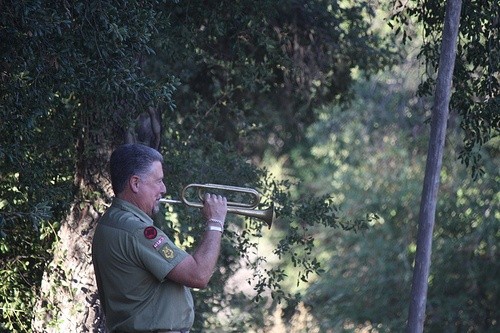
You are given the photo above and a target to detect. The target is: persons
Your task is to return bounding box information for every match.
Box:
[92,144,227,333]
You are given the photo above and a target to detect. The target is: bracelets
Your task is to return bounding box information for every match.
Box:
[205,225,224,233]
[205,218,223,224]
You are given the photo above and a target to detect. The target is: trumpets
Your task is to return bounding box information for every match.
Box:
[158,182,273,229]
